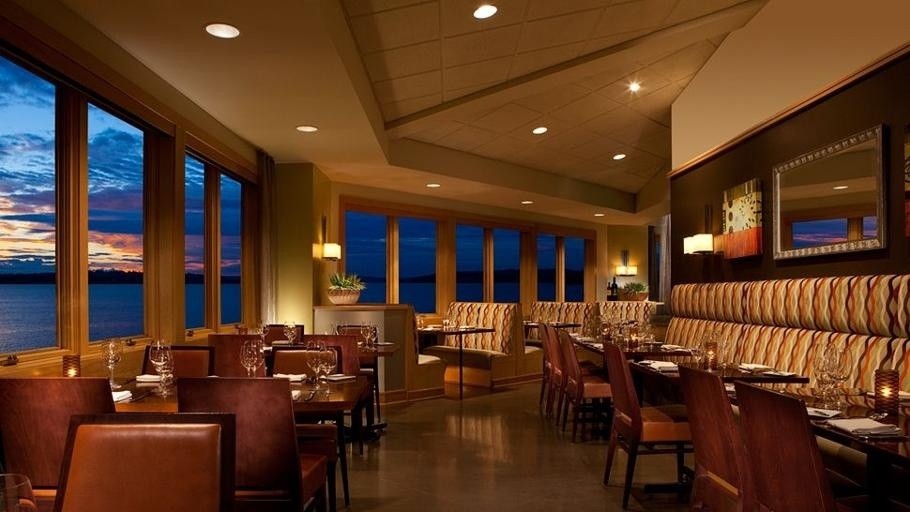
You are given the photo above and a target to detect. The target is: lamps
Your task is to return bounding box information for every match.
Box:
[874,368,900,416]
[602,328,611,344]
[705,342,718,370]
[683,233,714,258]
[616,265,638,277]
[323,243,342,262]
[63,354,82,377]
[628,327,639,349]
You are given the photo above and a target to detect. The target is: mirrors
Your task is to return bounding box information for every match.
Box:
[771,123,889,262]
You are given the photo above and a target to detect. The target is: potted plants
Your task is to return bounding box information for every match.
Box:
[618,282,647,301]
[324,271,369,306]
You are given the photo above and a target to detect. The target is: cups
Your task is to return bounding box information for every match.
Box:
[307,342,322,392]
[370,321,379,347]
[259,323,269,338]
[154,349,174,395]
[318,339,335,397]
[361,321,371,349]
[101,339,123,390]
[240,345,256,379]
[826,348,853,410]
[815,343,837,407]
[336,320,347,336]
[282,324,295,344]
[148,338,169,380]
[244,341,264,377]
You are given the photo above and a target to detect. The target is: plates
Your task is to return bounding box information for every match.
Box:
[807,407,842,419]
[320,373,356,381]
[763,370,797,376]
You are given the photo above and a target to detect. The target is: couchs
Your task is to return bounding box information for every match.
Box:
[516,302,544,375]
[422,302,517,390]
[663,274,910,401]
[527,300,597,362]
[407,304,446,405]
[594,301,657,344]
[594,303,605,337]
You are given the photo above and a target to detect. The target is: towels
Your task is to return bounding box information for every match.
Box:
[292,389,301,401]
[273,372,307,382]
[827,417,901,436]
[661,344,684,350]
[135,374,162,382]
[111,390,132,402]
[738,362,769,371]
[650,362,678,372]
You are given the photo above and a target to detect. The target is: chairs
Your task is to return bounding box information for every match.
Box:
[52,412,236,511]
[269,343,350,512]
[733,379,872,512]
[548,326,606,432]
[142,345,216,382]
[207,334,266,377]
[538,323,597,419]
[558,330,614,444]
[602,341,696,510]
[177,376,328,512]
[303,335,372,456]
[677,364,743,512]
[262,324,304,345]
[337,325,382,426]
[0,377,122,489]
